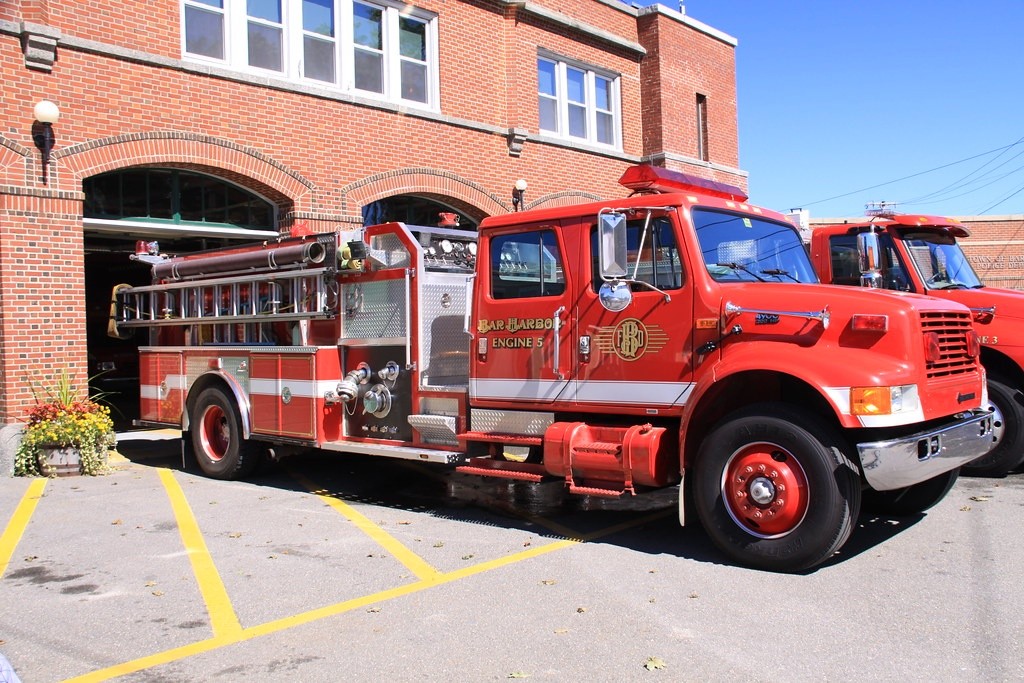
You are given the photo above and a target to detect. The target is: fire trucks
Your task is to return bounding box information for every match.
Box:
[623,212,1023,478]
[113,193,1006,571]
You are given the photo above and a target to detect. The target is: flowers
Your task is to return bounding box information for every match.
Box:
[13,360,118,480]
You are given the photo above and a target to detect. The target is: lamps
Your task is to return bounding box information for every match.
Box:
[33,100,61,163]
[512,178,527,211]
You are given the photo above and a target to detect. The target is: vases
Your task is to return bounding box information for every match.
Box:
[35,443,89,479]
